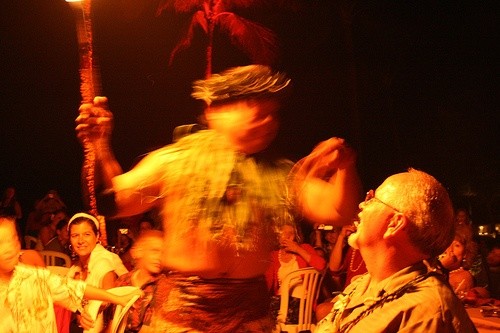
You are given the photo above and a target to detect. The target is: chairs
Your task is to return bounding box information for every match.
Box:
[272,267,328,333]
[82,285,141,333]
[38,250,72,267]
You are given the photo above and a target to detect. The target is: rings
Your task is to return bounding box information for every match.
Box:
[78,325,82,327]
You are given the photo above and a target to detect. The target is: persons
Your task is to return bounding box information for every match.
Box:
[440,207,500,306]
[26,188,163,333]
[0,209,146,333]
[267,220,370,323]
[0,186,24,220]
[74,65,361,333]
[310,170,479,333]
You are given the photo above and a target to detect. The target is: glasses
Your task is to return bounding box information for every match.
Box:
[365,189,400,213]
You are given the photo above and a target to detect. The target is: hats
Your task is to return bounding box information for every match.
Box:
[158,0,293,107]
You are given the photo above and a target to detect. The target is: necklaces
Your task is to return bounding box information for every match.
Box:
[448,266,464,274]
[349,246,365,272]
[131,268,141,289]
[75,253,91,281]
[276,248,302,285]
[330,273,425,333]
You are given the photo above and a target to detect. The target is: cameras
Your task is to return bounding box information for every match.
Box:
[119,228,128,235]
[317,225,333,230]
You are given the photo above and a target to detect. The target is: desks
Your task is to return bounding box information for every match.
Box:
[464,297,500,333]
